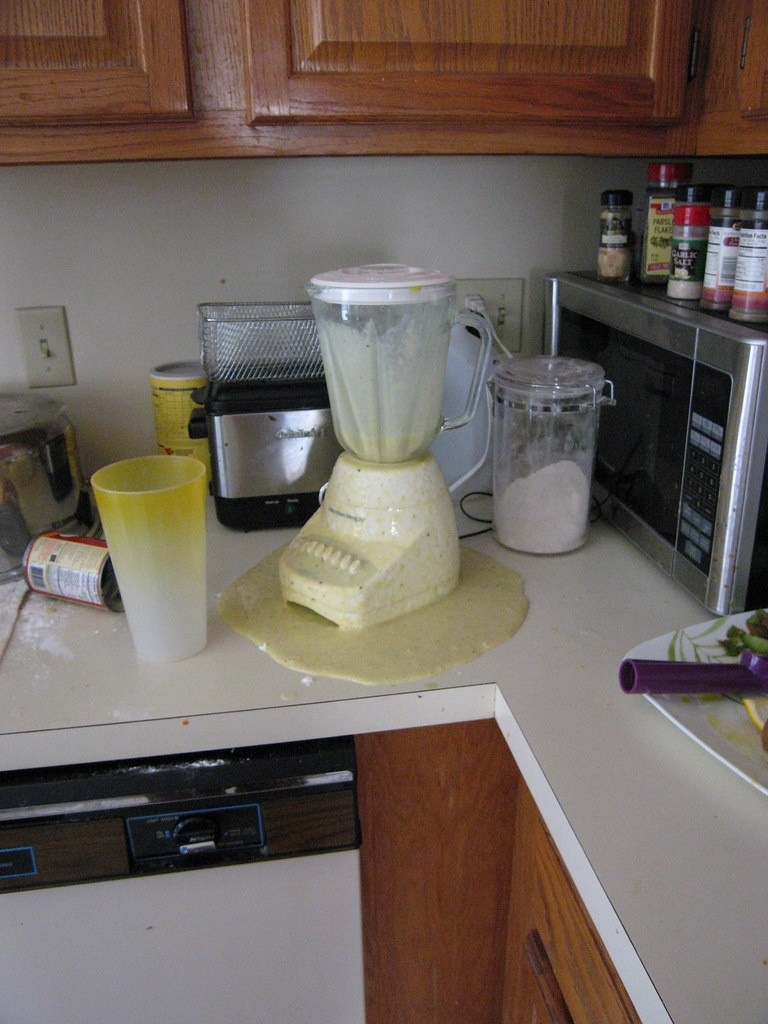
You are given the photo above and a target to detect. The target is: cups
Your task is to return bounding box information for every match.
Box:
[91,455,209,665]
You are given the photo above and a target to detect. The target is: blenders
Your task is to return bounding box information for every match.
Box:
[276,261,493,625]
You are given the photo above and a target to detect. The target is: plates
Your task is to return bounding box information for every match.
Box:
[620,608,768,798]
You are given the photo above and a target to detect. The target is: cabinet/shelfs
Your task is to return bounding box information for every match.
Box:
[508,778,642,1023]
[1,0,767,157]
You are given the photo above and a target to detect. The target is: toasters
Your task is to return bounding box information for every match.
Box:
[188,366,344,531]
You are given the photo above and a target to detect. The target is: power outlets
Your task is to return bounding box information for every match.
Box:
[456,280,523,356]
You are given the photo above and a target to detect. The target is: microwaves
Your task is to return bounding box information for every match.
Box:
[540,267,768,616]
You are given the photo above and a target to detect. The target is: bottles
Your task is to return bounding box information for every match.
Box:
[23,529,125,615]
[594,160,768,326]
[485,354,615,556]
[148,359,215,495]
